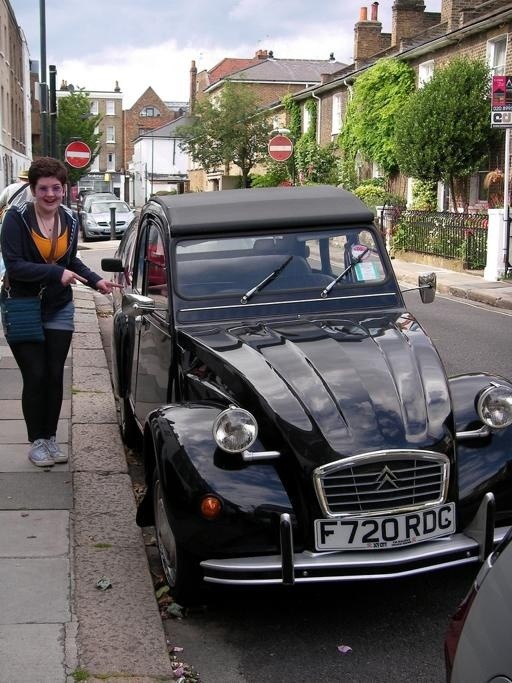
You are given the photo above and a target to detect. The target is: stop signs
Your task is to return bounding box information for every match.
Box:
[64,139,93,168]
[267,134,296,162]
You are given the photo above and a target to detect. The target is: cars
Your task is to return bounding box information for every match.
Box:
[104,211,167,317]
[76,187,137,242]
[446,526,512,683]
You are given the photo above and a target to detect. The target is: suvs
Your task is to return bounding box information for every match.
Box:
[106,179,512,611]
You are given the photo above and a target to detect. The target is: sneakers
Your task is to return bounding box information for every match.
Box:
[46,436,68,464]
[28,439,56,467]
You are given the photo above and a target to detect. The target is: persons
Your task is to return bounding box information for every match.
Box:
[0,156,124,467]
[0,169,34,228]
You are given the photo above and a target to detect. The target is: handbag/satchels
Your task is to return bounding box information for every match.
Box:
[0,204,8,224]
[1,295,48,345]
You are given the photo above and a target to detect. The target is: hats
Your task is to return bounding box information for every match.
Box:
[18,170,29,179]
